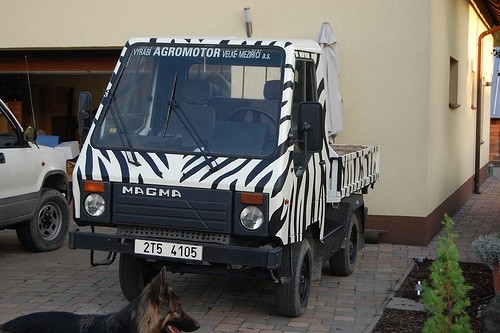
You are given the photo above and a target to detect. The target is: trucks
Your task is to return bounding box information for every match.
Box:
[70,34,380,316]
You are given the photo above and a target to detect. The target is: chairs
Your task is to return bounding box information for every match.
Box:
[163,79,281,148]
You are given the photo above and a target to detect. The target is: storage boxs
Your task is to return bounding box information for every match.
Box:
[55,141,80,159]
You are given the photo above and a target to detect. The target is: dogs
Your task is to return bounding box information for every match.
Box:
[0,266,202,333]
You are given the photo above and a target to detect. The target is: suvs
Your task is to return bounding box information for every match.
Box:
[0,99,66,252]
